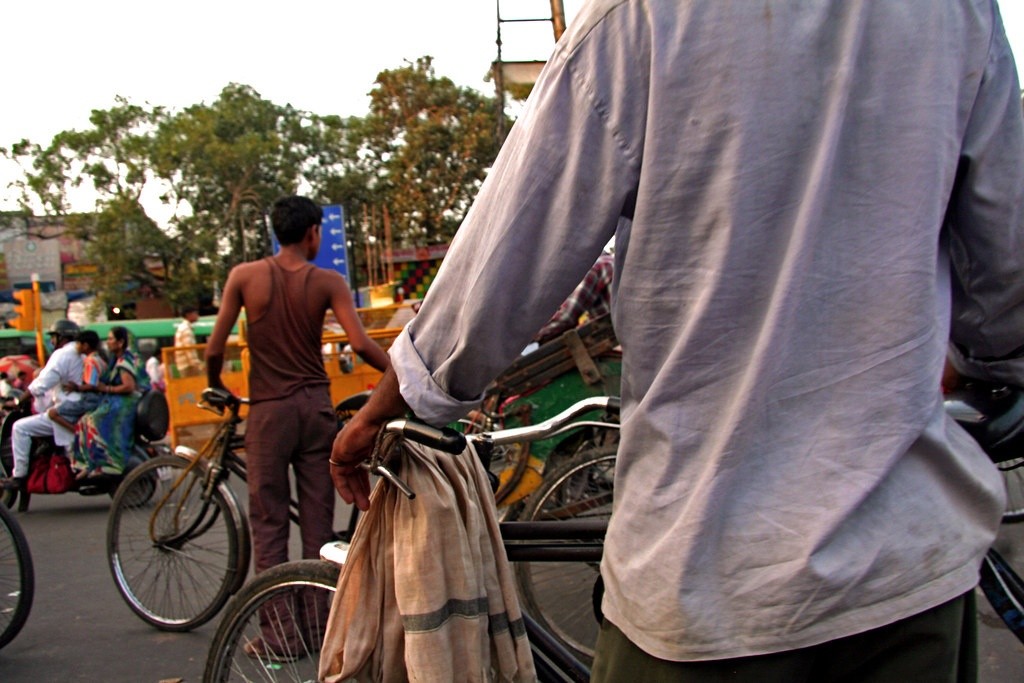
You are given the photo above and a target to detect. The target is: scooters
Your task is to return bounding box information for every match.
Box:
[0,366,169,508]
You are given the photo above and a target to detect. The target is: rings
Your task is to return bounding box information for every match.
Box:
[329,459,342,466]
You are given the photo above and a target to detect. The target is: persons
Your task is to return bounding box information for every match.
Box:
[202,196,391,662]
[0,319,163,490]
[531,247,614,344]
[173,306,200,378]
[328,0,1024,683]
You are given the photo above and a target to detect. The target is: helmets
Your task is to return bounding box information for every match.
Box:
[46,319,81,338]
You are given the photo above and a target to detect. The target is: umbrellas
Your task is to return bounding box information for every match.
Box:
[0,355,40,383]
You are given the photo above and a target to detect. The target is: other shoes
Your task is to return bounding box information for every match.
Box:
[0,476,26,487]
[243,638,298,661]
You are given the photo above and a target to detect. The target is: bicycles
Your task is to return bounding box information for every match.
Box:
[104,383,1024,683]
